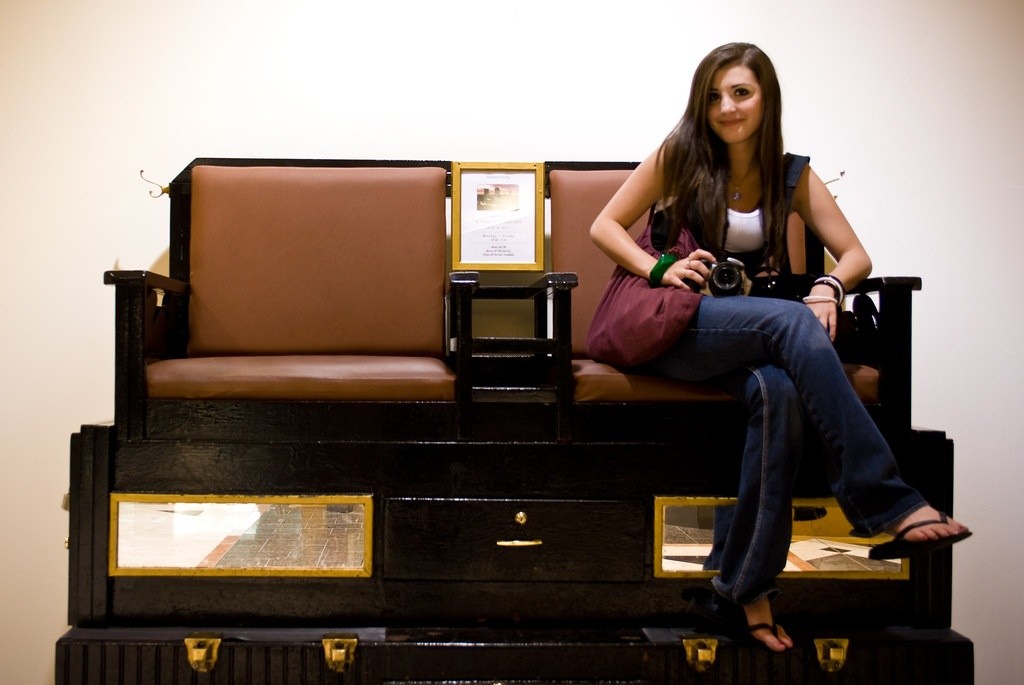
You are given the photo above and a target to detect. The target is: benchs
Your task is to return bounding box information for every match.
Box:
[104,157,921,445]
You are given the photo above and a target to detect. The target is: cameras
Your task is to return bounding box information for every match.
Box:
[690,258,754,297]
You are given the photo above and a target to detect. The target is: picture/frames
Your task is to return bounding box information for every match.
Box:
[451,161,546,271]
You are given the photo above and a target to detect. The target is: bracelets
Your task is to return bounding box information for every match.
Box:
[802,296,837,303]
[813,275,845,306]
[649,255,677,286]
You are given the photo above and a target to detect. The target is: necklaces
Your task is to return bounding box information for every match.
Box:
[729,170,750,201]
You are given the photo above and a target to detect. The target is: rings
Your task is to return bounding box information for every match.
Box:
[687,260,693,268]
[689,252,695,260]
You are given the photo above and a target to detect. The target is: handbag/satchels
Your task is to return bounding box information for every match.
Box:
[583,202,703,371]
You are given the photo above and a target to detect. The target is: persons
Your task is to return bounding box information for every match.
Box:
[589,42,970,654]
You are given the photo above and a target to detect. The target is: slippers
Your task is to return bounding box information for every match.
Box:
[710,589,801,656]
[867,510,973,560]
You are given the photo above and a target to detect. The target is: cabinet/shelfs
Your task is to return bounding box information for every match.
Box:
[54,422,975,684]
